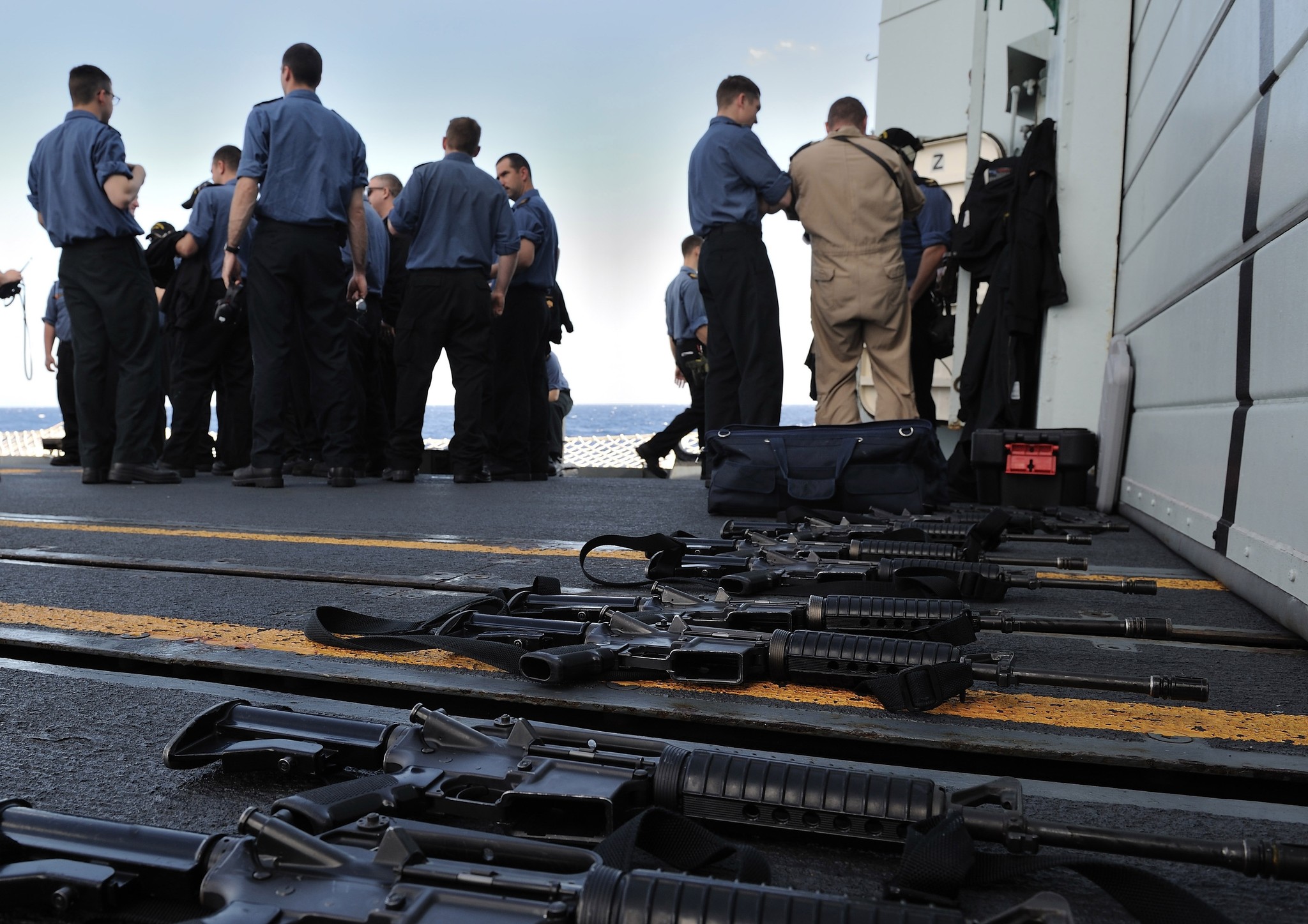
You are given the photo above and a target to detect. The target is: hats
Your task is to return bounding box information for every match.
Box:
[146,222,176,239]
[878,128,915,149]
[182,181,213,209]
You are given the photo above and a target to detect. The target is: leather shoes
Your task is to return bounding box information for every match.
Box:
[700,474,711,487]
[51,452,565,489]
[636,443,669,480]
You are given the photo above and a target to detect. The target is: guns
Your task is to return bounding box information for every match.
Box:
[0,525,1307,923]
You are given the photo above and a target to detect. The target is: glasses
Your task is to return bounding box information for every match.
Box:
[367,187,383,196]
[97,91,120,105]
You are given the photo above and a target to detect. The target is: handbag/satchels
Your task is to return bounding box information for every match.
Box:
[694,419,940,517]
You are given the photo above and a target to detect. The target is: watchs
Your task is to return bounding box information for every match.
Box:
[223,242,241,255]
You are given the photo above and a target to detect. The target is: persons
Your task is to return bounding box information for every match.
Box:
[40,280,84,468]
[162,179,234,460]
[874,128,955,435]
[382,116,522,484]
[145,145,256,474]
[221,44,372,489]
[362,174,406,478]
[634,235,705,479]
[26,65,183,484]
[133,222,181,310]
[335,192,391,478]
[0,269,22,288]
[687,74,792,483]
[787,95,922,423]
[486,152,558,481]
[541,351,574,477]
